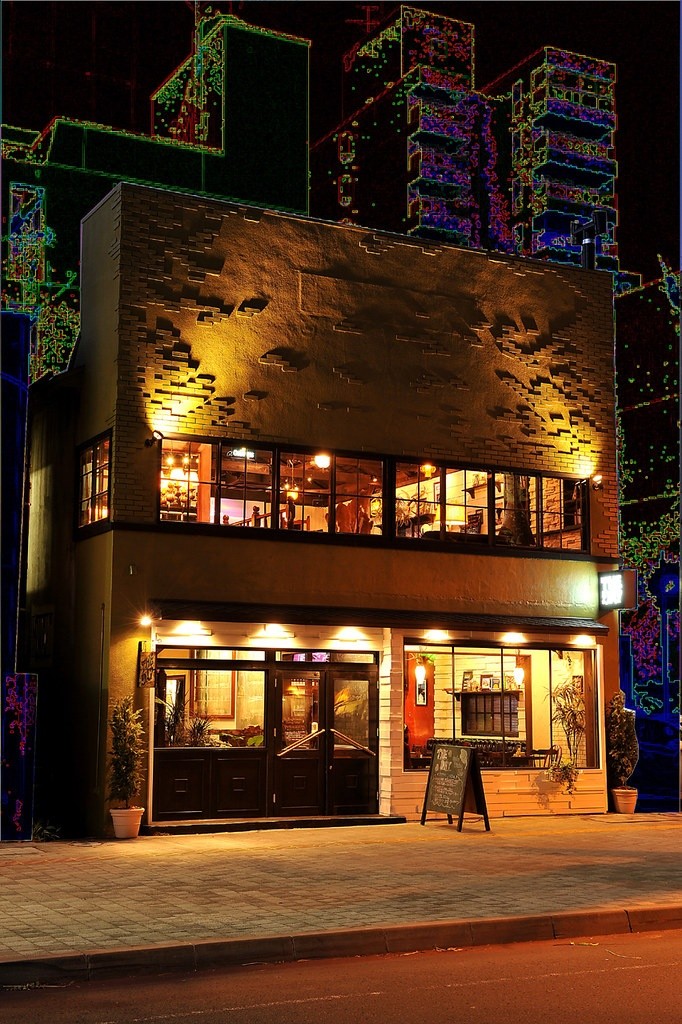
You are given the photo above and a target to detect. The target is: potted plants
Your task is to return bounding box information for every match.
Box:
[105,697,148,838]
[608,689,639,813]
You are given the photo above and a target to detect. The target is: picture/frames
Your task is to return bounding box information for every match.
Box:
[479,674,517,691]
[433,482,440,510]
[494,496,505,531]
[416,679,427,705]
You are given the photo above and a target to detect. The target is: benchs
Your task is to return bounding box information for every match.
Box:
[427,738,527,768]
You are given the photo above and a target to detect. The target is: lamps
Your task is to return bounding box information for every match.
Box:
[144,430,164,447]
[593,475,604,490]
[420,464,436,477]
[314,456,331,468]
[513,668,524,692]
[415,665,426,684]
[287,489,298,500]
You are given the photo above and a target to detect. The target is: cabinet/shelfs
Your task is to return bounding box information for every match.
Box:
[280,653,368,750]
[462,481,503,499]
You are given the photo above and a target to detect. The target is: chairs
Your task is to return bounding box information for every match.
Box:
[543,745,563,768]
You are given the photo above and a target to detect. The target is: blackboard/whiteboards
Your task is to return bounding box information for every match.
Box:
[426,744,471,815]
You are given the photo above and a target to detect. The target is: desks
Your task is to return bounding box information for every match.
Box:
[410,755,432,770]
[488,751,545,768]
[374,513,436,538]
[436,520,468,532]
